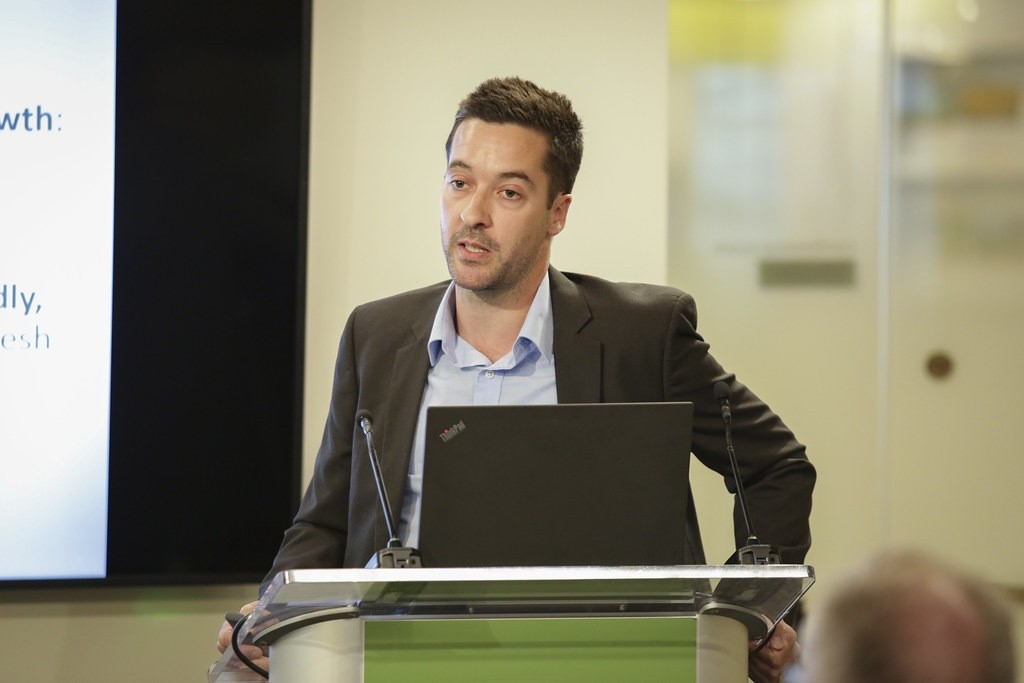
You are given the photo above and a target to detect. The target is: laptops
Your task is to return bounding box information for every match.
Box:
[421,401,694,567]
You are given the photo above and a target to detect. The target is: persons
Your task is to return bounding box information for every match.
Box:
[821,547,1018,683]
[217,76,817,683]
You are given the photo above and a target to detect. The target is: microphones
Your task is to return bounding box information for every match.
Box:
[713,380,785,606]
[356,410,421,609]
